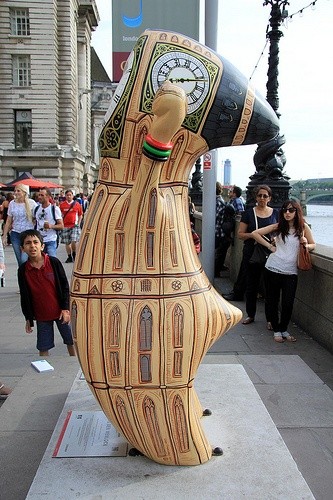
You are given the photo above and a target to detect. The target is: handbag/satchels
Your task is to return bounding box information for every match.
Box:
[298,228,312,271]
[247,242,275,269]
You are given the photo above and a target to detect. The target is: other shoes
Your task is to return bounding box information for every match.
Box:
[65,257,72,263]
[273,336,283,342]
[72,252,75,259]
[242,316,254,324]
[215,273,220,278]
[268,322,273,330]
[256,295,264,303]
[283,336,296,342]
[222,294,243,301]
[221,266,228,271]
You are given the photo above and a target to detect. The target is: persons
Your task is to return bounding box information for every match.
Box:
[252,197,315,342]
[0,184,95,287]
[17,229,77,356]
[187,182,280,329]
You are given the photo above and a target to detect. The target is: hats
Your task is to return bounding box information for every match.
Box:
[216,182,221,190]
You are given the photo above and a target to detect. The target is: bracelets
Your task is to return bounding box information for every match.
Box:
[269,244,273,250]
[142,134,174,162]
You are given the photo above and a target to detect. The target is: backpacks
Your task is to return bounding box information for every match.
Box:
[190,222,201,253]
[218,200,236,233]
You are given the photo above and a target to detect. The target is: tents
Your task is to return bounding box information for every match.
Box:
[0,172,55,192]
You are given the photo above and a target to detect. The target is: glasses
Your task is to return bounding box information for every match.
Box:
[282,208,296,213]
[257,194,269,198]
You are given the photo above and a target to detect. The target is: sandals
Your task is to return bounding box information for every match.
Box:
[0,384,14,399]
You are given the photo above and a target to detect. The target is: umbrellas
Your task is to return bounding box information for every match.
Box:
[13,177,65,200]
